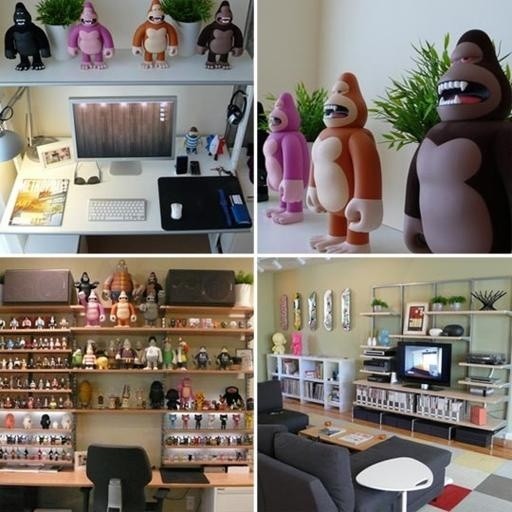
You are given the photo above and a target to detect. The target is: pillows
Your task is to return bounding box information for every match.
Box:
[272,431,355,511]
[258,423,287,455]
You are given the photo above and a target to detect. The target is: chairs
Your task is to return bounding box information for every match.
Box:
[82,444,164,512]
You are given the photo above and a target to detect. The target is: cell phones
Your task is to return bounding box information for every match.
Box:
[191,161,200,175]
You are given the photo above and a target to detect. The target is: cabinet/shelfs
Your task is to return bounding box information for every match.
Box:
[358,310,511,392]
[70,306,164,413]
[164,308,253,469]
[265,351,355,414]
[0,306,72,472]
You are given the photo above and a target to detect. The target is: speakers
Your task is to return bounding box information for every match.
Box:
[4,268,74,304]
[175,156,188,174]
[164,269,235,307]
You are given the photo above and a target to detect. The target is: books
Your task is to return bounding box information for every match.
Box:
[281,378,323,401]
[1,463,42,472]
[315,426,345,437]
[40,462,65,473]
[8,178,70,227]
[338,430,375,445]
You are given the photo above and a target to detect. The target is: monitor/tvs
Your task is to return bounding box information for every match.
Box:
[69,97,175,175]
[397,342,452,392]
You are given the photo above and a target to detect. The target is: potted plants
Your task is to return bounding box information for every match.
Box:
[429,295,448,311]
[234,268,254,305]
[445,295,465,311]
[370,299,389,312]
[260,77,331,154]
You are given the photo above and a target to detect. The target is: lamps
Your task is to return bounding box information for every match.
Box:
[1,86,54,166]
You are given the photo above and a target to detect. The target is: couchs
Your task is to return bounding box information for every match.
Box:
[259,380,452,511]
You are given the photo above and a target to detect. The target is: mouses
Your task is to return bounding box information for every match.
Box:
[169,202,183,220]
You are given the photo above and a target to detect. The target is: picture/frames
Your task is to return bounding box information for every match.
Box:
[37,139,76,168]
[73,450,87,471]
[402,300,429,336]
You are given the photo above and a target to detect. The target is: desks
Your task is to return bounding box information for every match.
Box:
[1,469,254,511]
[353,457,432,511]
[0,135,252,254]
[353,380,512,449]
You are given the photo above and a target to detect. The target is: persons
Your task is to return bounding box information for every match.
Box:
[401,29,512,256]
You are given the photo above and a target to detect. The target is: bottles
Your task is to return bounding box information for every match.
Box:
[97,383,147,408]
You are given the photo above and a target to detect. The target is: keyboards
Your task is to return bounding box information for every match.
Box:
[88,199,146,221]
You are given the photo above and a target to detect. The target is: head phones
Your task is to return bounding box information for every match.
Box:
[227,89,247,126]
